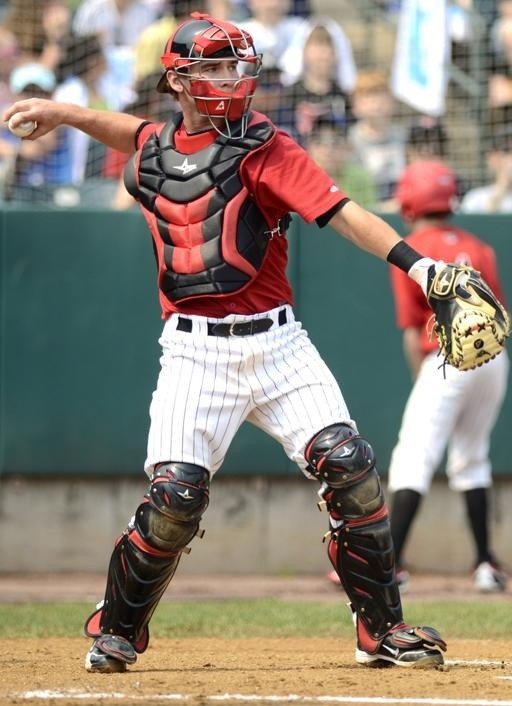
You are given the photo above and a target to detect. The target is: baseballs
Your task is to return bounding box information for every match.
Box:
[8,111,35,137]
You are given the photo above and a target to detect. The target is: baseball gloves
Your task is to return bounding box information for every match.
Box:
[426,262,510,379]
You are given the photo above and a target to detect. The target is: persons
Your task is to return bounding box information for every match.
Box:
[1,11,509,675]
[0,0,512,215]
[325,156,507,595]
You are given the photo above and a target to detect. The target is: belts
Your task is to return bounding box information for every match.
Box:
[176,309,288,337]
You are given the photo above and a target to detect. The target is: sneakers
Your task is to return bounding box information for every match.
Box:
[355,639,444,668]
[330,567,410,594]
[473,562,505,592]
[84,636,127,672]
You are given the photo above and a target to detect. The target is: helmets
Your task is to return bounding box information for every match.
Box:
[160,12,253,69]
[396,161,457,215]
